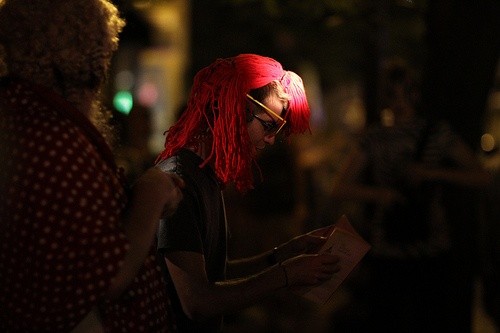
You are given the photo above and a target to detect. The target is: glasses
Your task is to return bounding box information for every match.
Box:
[246,114,276,134]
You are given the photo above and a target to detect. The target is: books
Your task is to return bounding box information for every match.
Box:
[297,214,373,308]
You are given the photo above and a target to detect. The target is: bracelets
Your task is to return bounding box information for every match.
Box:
[279,262,291,288]
[273,246,282,262]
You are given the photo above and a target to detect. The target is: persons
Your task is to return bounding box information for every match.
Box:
[138,52,341,333]
[1,0,186,333]
